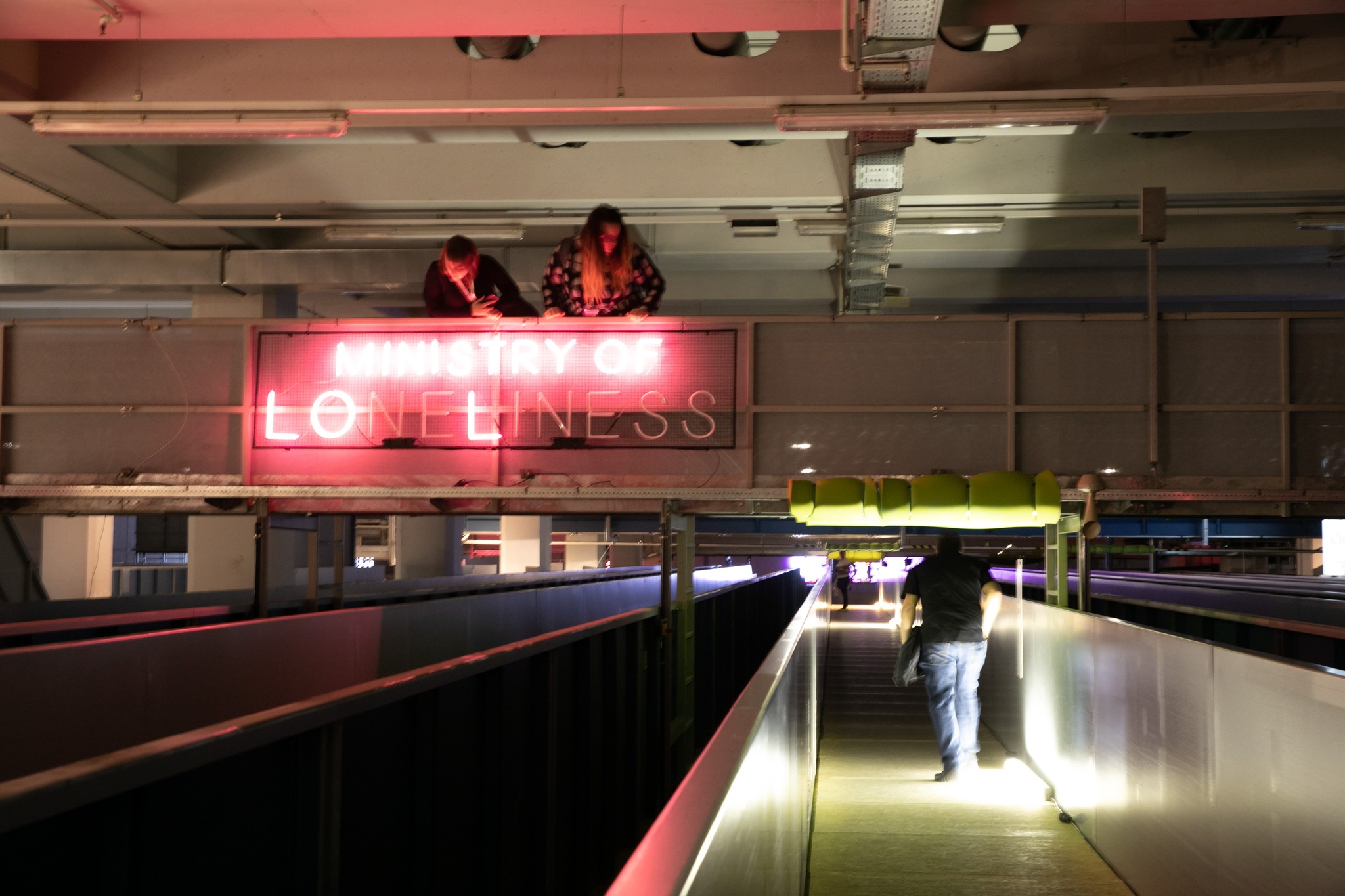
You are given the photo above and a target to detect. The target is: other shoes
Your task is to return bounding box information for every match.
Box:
[837,608,849,611]
[934,758,962,781]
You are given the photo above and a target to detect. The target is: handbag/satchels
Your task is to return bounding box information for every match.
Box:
[836,564,850,575]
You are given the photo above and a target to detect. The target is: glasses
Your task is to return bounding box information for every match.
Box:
[599,232,617,243]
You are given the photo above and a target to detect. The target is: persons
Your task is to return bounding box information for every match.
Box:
[424,234,541,323]
[835,550,857,611]
[899,532,1002,782]
[542,205,666,323]
